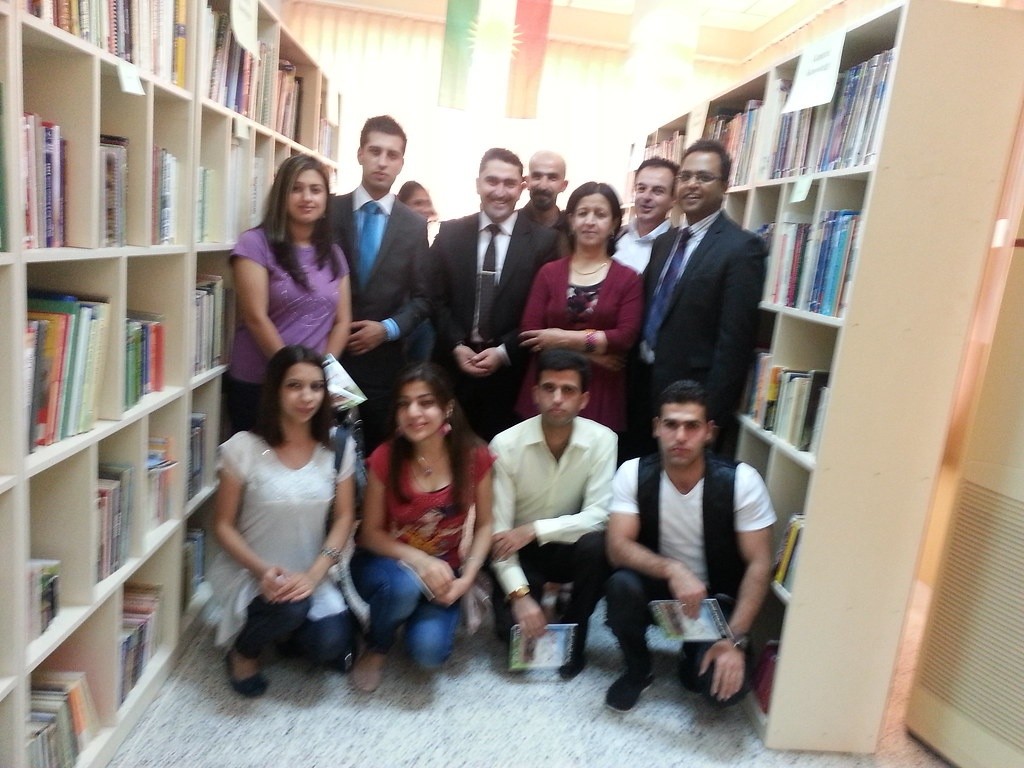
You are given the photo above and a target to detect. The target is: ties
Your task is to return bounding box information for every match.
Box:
[356,202,381,293]
[477,224,501,343]
[645,228,691,350]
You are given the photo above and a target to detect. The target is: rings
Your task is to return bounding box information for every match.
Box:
[682,603,686,606]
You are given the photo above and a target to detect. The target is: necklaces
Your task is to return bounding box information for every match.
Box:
[423,467,433,476]
[574,263,607,275]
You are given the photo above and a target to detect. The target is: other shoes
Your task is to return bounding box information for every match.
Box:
[604,668,656,713]
[559,652,589,681]
[351,654,384,694]
[224,647,265,700]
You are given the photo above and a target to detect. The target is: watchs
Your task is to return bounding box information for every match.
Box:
[733,633,749,650]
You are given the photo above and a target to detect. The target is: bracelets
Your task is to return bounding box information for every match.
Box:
[510,586,530,599]
[321,547,340,564]
[584,329,597,352]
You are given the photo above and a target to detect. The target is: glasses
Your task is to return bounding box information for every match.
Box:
[675,171,724,183]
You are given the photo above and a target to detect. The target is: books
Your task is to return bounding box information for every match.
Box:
[508,623,578,670]
[643,49,892,452]
[397,559,435,602]
[22,0,304,768]
[649,599,736,641]
[751,512,804,711]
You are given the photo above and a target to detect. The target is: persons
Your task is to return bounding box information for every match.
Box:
[518,150,568,229]
[604,379,777,714]
[611,157,680,275]
[329,115,433,454]
[488,348,618,679]
[354,360,499,691]
[620,138,765,465]
[515,182,644,433]
[429,147,563,444]
[205,343,357,697]
[228,153,352,435]
[398,180,439,221]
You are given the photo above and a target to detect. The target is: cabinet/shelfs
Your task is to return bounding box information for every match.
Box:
[0,1,342,768]
[620,1,1024,754]
